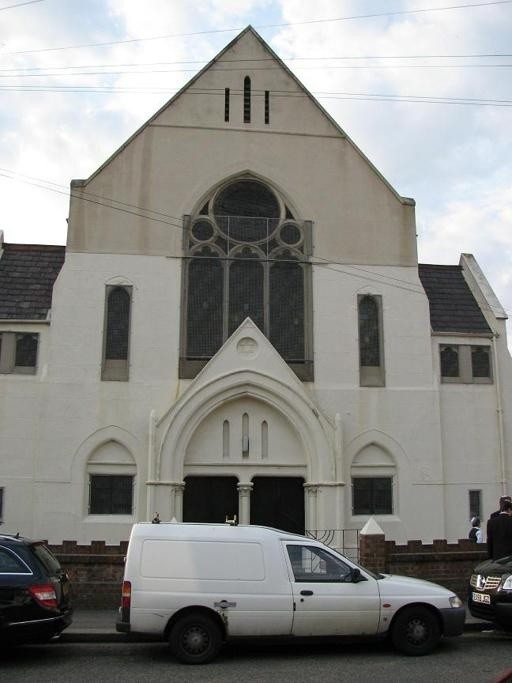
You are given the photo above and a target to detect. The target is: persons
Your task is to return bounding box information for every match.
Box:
[469,517,484,543]
[487,499,512,561]
[490,496,512,519]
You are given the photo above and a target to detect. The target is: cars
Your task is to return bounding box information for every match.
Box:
[467,539,512,631]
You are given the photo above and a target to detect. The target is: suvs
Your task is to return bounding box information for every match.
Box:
[0,530,78,653]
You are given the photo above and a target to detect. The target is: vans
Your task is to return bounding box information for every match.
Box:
[112,513,468,654]
